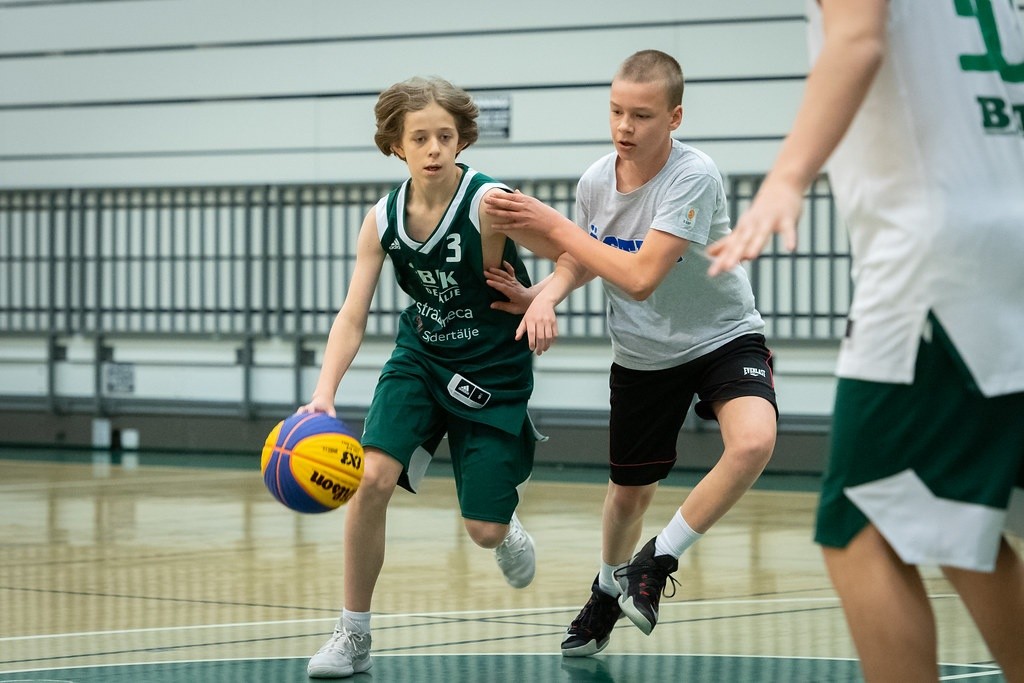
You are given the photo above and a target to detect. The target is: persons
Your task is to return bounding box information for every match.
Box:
[295,77,587,677]
[707,1,1024,683]
[484,49,780,655]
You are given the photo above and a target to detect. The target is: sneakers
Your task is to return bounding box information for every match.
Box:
[492,511,536,589]
[559,572,627,656]
[306,613,373,676]
[611,536,681,636]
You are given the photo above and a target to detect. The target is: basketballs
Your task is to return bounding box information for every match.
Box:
[260,411,366,515]
